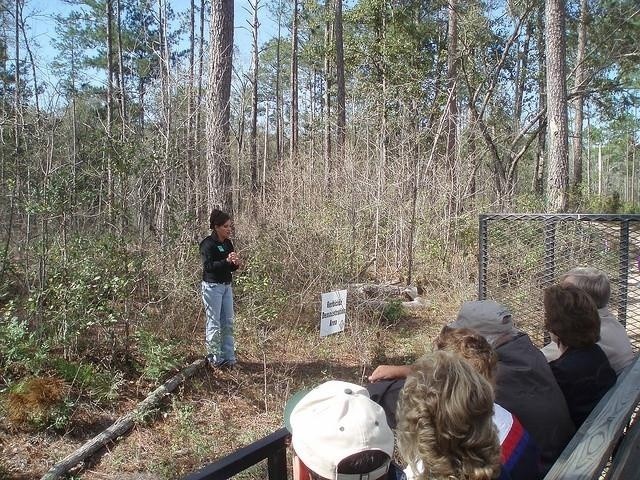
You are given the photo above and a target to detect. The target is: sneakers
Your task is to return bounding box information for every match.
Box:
[216,358,241,370]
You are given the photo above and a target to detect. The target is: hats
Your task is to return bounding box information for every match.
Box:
[451,300,513,335]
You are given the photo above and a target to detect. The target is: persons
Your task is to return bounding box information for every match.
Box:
[540,268,633,376]
[367,298,576,463]
[394,350,542,480]
[435,323,498,395]
[543,281,617,429]
[200,209,240,370]
